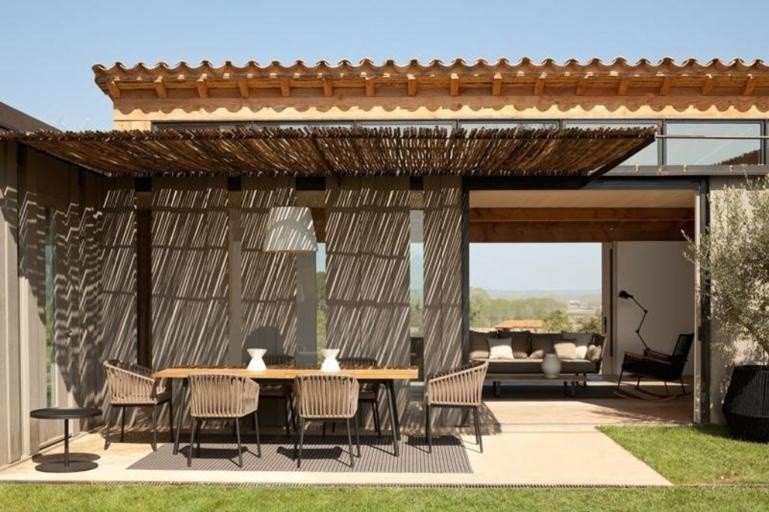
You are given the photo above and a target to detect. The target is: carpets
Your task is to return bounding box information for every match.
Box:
[124,435,473,474]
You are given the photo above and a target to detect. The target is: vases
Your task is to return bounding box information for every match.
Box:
[541,354,562,378]
[245,348,341,371]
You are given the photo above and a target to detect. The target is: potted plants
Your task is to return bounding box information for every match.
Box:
[681,163,769,443]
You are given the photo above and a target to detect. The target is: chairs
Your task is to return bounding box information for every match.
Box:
[185,371,263,469]
[613,334,694,403]
[332,356,380,435]
[289,373,364,468]
[104,355,174,452]
[244,355,297,432]
[422,356,488,454]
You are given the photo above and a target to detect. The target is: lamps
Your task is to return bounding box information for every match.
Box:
[262,164,318,253]
[617,289,650,379]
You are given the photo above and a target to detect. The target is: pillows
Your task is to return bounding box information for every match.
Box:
[468,330,603,361]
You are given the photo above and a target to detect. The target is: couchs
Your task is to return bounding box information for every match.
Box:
[469,329,607,399]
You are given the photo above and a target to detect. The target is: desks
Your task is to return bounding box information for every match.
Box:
[150,363,419,459]
[484,375,589,397]
[30,408,102,473]
